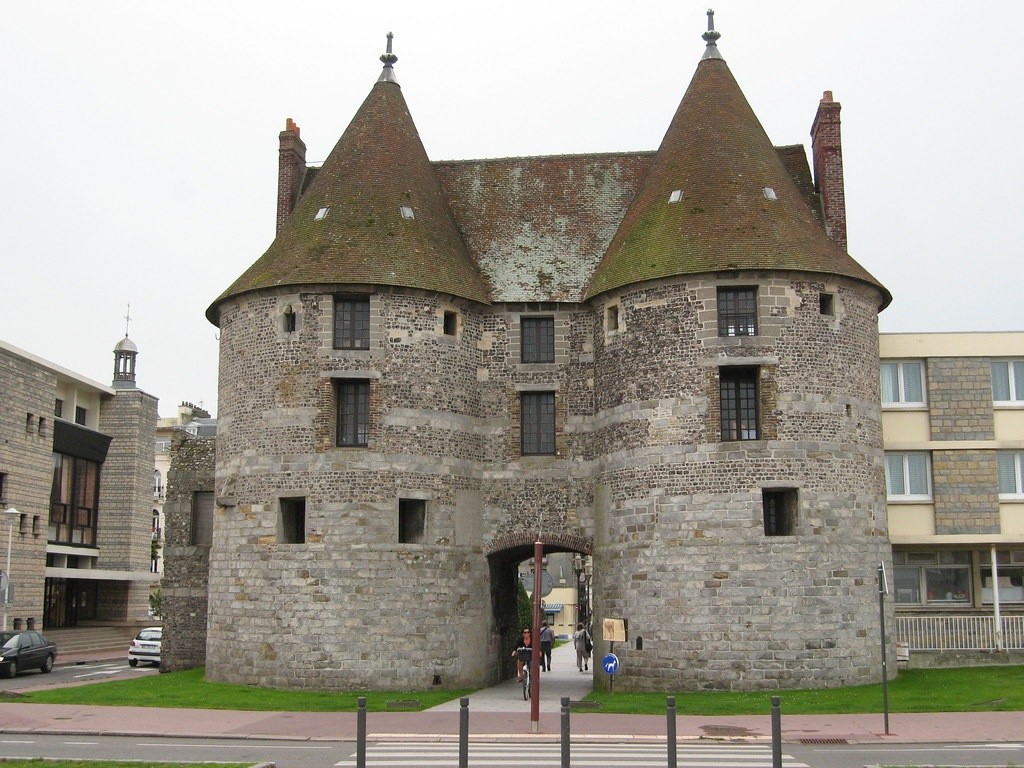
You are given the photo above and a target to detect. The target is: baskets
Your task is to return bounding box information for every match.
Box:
[517,648,532,661]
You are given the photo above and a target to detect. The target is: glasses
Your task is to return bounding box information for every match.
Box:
[523,632,529,634]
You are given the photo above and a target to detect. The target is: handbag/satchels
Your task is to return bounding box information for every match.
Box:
[584,643,593,653]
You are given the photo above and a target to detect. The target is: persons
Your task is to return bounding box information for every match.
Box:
[574,623,591,672]
[540,620,555,672]
[511,628,542,682]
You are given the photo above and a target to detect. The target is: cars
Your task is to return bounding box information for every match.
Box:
[0,630,56,678]
[126,627,162,666]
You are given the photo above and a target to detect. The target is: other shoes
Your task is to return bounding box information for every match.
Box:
[547,666,550,671]
[542,667,545,672]
[585,667,588,670]
[579,670,583,672]
[517,678,523,682]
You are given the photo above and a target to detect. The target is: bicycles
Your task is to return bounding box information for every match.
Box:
[513,648,532,700]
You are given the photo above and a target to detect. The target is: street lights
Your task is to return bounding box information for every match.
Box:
[528,541,546,736]
[585,574,592,635]
[3,509,21,629]
